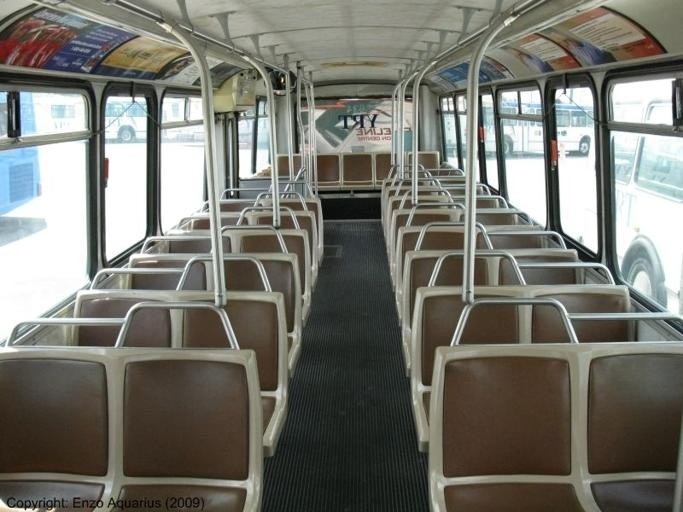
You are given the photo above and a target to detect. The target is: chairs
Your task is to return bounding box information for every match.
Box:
[1,151,683,512]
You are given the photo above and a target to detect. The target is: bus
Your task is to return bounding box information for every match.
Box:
[0,93,42,219]
[250,102,466,157]
[476,102,595,156]
[80,101,204,141]
[577,80,683,324]
